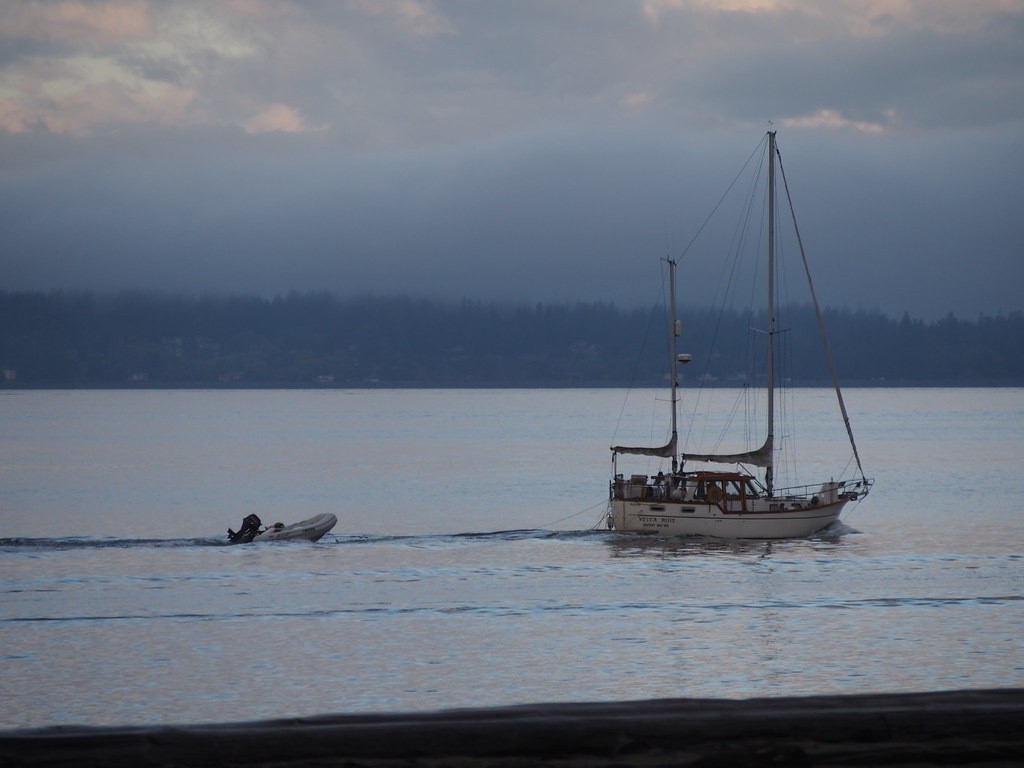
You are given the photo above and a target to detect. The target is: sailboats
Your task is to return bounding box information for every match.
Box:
[605,118,875,539]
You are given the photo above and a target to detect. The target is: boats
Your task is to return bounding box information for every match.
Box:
[227,512,337,545]
